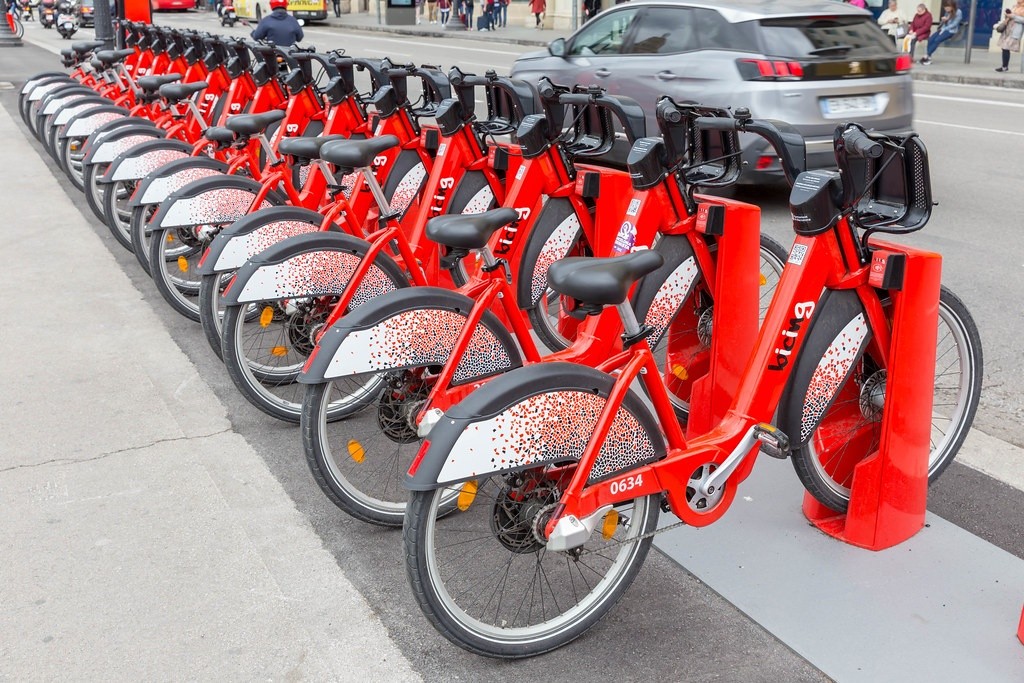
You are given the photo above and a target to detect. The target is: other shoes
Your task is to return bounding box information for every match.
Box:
[995,67,1009,72]
[923,59,932,65]
[918,57,927,63]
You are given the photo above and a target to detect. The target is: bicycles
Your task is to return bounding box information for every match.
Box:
[295,83,790,529]
[404,107,984,661]
[16,18,616,423]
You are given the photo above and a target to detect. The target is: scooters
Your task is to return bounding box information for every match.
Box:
[216,4,239,27]
[38,5,80,40]
[19,0,32,21]
[237,18,304,42]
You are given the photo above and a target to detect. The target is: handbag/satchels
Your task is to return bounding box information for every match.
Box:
[896,20,909,38]
[996,18,1010,33]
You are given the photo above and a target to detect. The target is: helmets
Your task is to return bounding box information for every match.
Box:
[269,0,288,11]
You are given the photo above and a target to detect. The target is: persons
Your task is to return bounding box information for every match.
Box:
[331,0,340,17]
[6,0,74,30]
[217,0,233,21]
[583,0,601,19]
[877,0,905,48]
[902,3,933,63]
[917,0,963,65]
[417,0,511,32]
[842,0,866,9]
[528,0,547,30]
[614,0,631,38]
[249,0,304,55]
[995,0,1024,72]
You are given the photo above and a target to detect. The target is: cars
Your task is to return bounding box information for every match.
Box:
[79,1,117,28]
[511,1,917,181]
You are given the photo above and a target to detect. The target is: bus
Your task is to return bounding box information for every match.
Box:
[150,1,196,12]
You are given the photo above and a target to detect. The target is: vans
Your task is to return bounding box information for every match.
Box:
[233,0,325,24]
